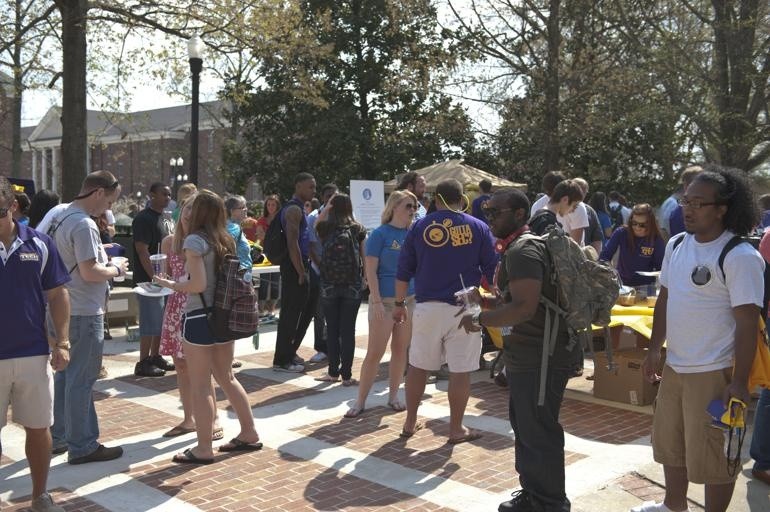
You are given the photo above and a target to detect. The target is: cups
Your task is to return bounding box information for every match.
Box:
[646,297,658,308]
[111,257,125,282]
[149,254,167,282]
[454,286,482,319]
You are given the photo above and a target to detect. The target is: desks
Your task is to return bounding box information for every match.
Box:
[251,265,282,327]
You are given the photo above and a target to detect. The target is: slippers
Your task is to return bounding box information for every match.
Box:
[212,415,224,440]
[343,407,364,418]
[313,372,341,382]
[400,421,427,437]
[217,438,264,452]
[447,428,484,445]
[387,400,406,412]
[162,425,197,438]
[342,378,360,386]
[170,447,216,464]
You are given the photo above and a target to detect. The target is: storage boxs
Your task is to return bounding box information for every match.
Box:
[595,346,667,407]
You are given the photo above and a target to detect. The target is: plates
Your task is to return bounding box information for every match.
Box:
[636,271,660,277]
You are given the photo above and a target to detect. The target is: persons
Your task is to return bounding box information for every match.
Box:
[225,194,252,368]
[133,182,175,376]
[343,190,420,417]
[669,167,704,235]
[171,189,262,466]
[587,193,612,242]
[395,172,426,232]
[393,179,498,444]
[530,181,583,235]
[531,172,589,244]
[599,204,664,298]
[177,184,199,218]
[36,172,129,464]
[631,166,770,511]
[154,194,224,442]
[257,196,281,241]
[272,173,317,373]
[26,188,60,232]
[305,184,336,356]
[313,193,368,385]
[471,180,495,230]
[0,176,71,512]
[240,209,257,244]
[608,192,633,227]
[8,193,31,226]
[455,187,585,511]
[750,194,769,482]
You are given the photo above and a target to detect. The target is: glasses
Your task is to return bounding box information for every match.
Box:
[401,203,418,211]
[630,221,651,228]
[677,199,718,210]
[232,206,245,211]
[0,204,12,219]
[482,207,513,221]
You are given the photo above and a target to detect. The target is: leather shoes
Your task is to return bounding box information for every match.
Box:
[152,354,175,371]
[69,444,123,465]
[51,446,67,454]
[496,489,569,512]
[751,470,769,485]
[134,356,166,377]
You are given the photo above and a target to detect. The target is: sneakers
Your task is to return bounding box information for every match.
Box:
[310,351,328,363]
[232,358,243,369]
[270,314,280,325]
[273,362,305,373]
[631,500,689,512]
[259,315,275,325]
[31,491,66,512]
[292,354,305,364]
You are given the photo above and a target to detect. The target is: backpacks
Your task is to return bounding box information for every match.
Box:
[264,202,306,264]
[184,228,258,342]
[504,225,621,405]
[315,220,367,289]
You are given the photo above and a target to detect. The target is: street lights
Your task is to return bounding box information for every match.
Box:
[187,31,207,183]
[177,173,189,184]
[169,152,183,192]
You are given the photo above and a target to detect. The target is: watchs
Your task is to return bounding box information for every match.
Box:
[54,337,70,350]
[395,299,406,307]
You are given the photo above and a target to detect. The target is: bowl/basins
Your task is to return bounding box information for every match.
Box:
[619,296,635,305]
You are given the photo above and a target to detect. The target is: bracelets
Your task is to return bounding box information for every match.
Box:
[471,312,482,327]
[107,262,121,273]
